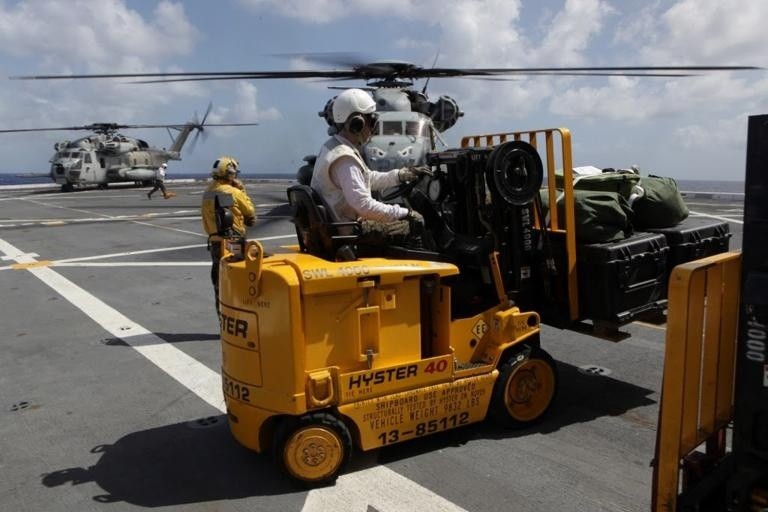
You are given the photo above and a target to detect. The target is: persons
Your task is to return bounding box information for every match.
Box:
[311,87,431,249]
[146,162,173,200]
[202,156,257,319]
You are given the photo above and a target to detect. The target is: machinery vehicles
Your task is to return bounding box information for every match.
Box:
[215,127,667,485]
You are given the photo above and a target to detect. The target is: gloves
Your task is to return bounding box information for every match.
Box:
[397,165,435,186]
[402,208,425,235]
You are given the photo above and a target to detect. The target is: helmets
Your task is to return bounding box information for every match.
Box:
[331,88,378,126]
[211,156,240,180]
